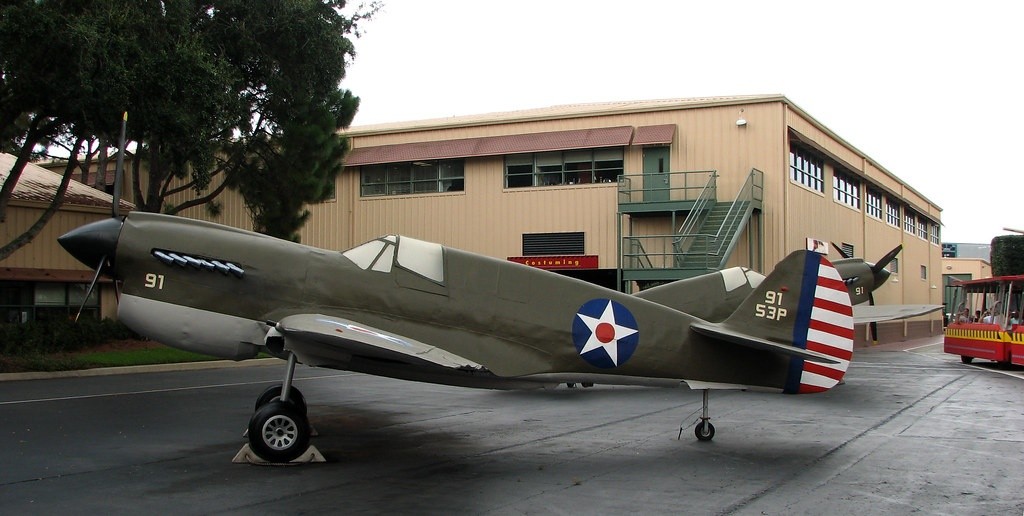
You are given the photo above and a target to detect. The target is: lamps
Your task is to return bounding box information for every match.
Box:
[736,108,747,126]
[947,265,951,269]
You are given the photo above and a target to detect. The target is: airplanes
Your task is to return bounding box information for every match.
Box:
[55,112,905,465]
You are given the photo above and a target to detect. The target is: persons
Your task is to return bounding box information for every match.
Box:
[973,307,1024,325]
[957,307,970,323]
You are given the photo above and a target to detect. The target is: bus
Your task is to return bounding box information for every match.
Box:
[944,276,1024,371]
